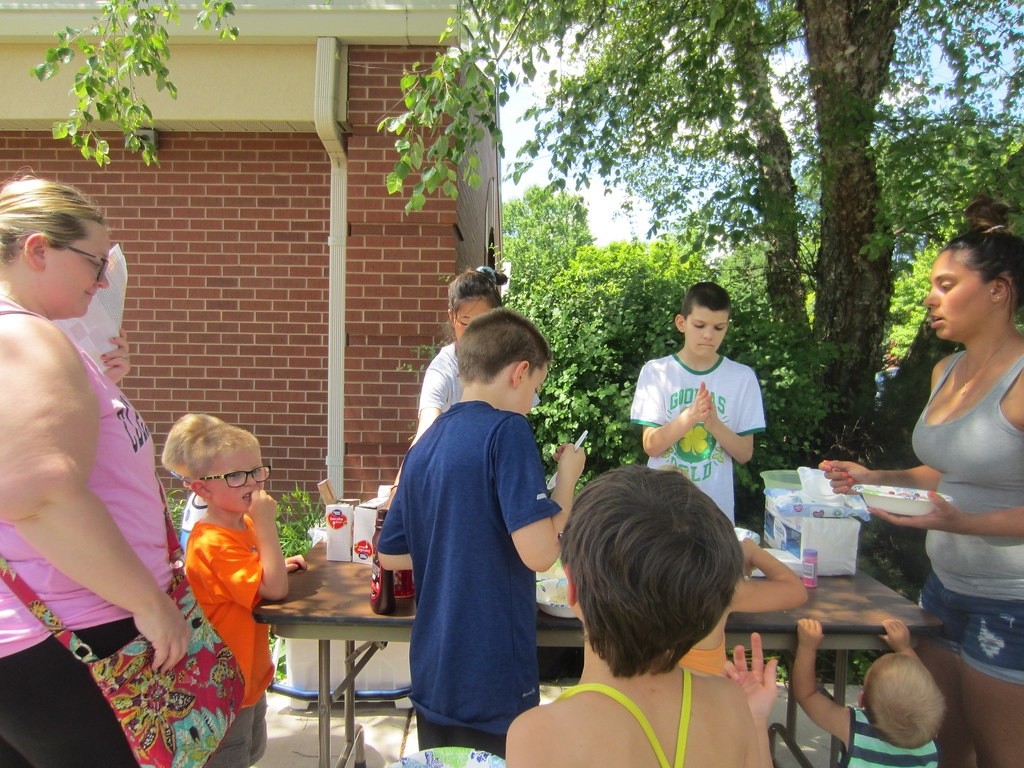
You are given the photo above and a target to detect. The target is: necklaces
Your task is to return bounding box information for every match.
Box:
[0,294,25,307]
[961,333,1015,395]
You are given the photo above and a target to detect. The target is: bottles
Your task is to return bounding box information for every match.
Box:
[802,548,818,588]
[369,509,396,614]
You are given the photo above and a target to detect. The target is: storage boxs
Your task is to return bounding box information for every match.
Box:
[316,479,360,561]
[353,497,388,563]
[764,495,860,575]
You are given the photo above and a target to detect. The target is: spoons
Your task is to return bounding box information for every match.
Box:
[547,430,588,490]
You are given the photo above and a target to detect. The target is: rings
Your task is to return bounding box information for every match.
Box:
[125,356,128,359]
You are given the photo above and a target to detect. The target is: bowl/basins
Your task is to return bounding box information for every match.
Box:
[536,578,578,619]
[852,485,954,515]
[387,746,505,768]
[734,527,761,547]
[760,469,803,490]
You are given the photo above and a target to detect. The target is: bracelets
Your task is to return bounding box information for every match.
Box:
[391,485,398,491]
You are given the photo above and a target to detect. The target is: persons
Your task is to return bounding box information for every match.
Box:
[792,619,946,768]
[679,539,808,676]
[185,425,308,768]
[0,177,192,768]
[630,282,766,528]
[382,267,542,510]
[506,465,778,768]
[162,413,224,553]
[819,192,1024,768]
[378,307,586,759]
[874,341,902,394]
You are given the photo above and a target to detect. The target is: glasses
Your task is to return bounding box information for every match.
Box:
[197,464,272,488]
[51,241,109,282]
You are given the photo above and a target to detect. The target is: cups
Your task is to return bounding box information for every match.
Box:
[394,569,415,600]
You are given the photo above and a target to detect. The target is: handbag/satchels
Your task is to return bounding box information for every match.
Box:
[89,575,245,768]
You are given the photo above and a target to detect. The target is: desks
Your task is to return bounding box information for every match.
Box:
[252,537,943,768]
